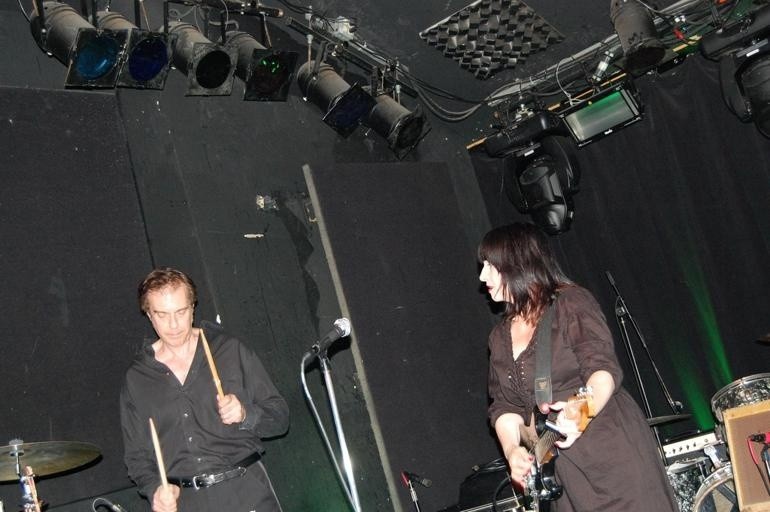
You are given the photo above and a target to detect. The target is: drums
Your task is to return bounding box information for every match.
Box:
[663,459,717,512]
[708,371,770,456]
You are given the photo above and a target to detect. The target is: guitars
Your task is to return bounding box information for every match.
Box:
[494,385,595,511]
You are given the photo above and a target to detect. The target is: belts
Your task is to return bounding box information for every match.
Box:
[165,453,262,490]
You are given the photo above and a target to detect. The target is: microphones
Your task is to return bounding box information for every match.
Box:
[407,470,433,489]
[313,314,354,362]
[674,398,684,414]
[746,432,770,446]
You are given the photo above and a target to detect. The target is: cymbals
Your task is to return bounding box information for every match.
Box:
[645,414,690,425]
[1,440,103,483]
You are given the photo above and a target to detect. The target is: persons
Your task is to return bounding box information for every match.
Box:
[119,267,291,512]
[477,220,682,512]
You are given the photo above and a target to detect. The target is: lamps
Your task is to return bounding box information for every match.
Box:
[3,0,429,164]
[606,0,667,78]
